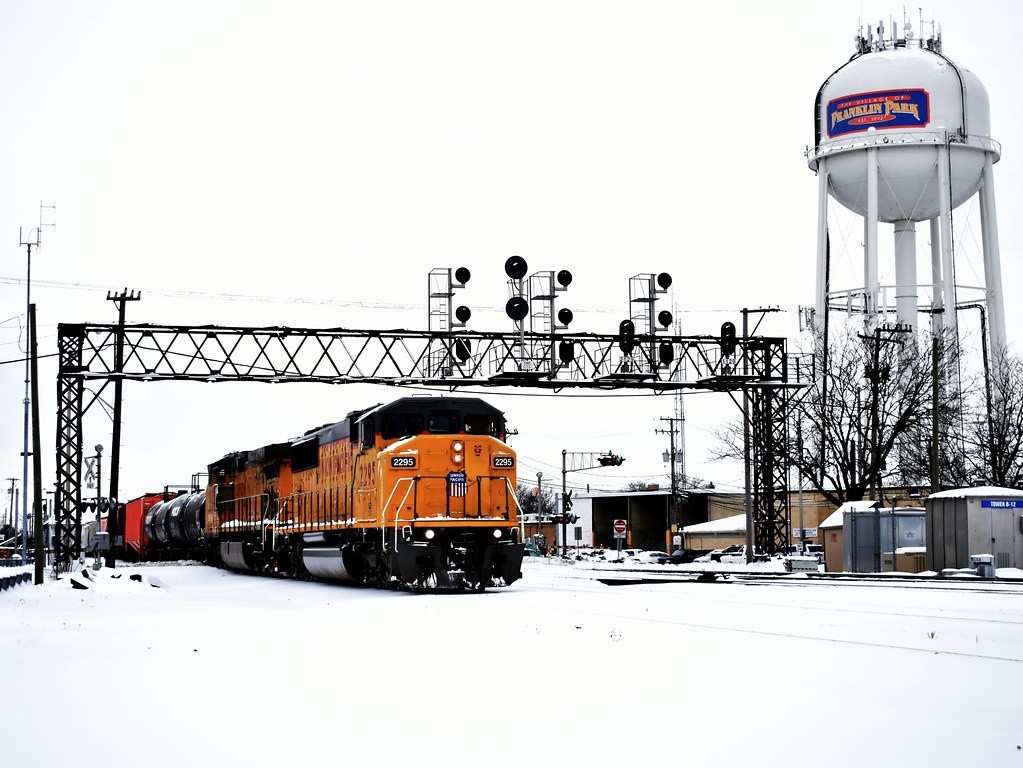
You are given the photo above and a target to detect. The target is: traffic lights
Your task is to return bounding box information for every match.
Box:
[597,456,626,466]
[549,512,579,524]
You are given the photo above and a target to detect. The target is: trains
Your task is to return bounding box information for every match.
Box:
[79,392,523,591]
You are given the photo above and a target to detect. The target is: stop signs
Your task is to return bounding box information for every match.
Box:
[613,518,627,538]
